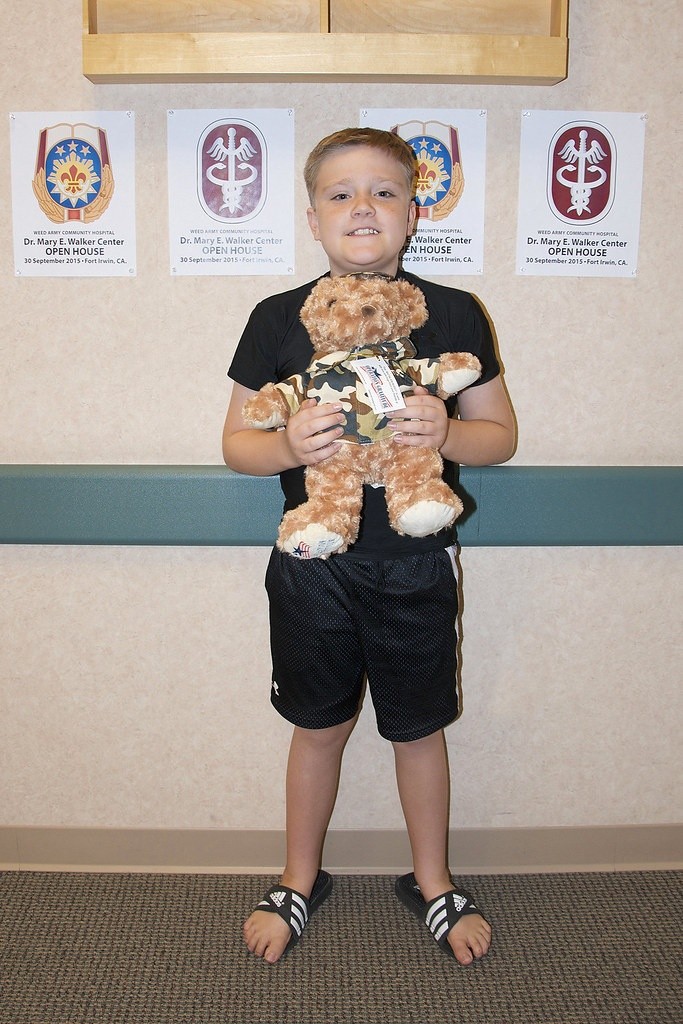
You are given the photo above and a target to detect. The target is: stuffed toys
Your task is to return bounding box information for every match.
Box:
[242,271,482,559]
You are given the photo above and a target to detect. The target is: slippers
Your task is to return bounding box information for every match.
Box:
[395,868,481,955]
[244,871,333,950]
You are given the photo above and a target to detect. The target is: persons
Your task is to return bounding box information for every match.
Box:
[221,128,519,965]
